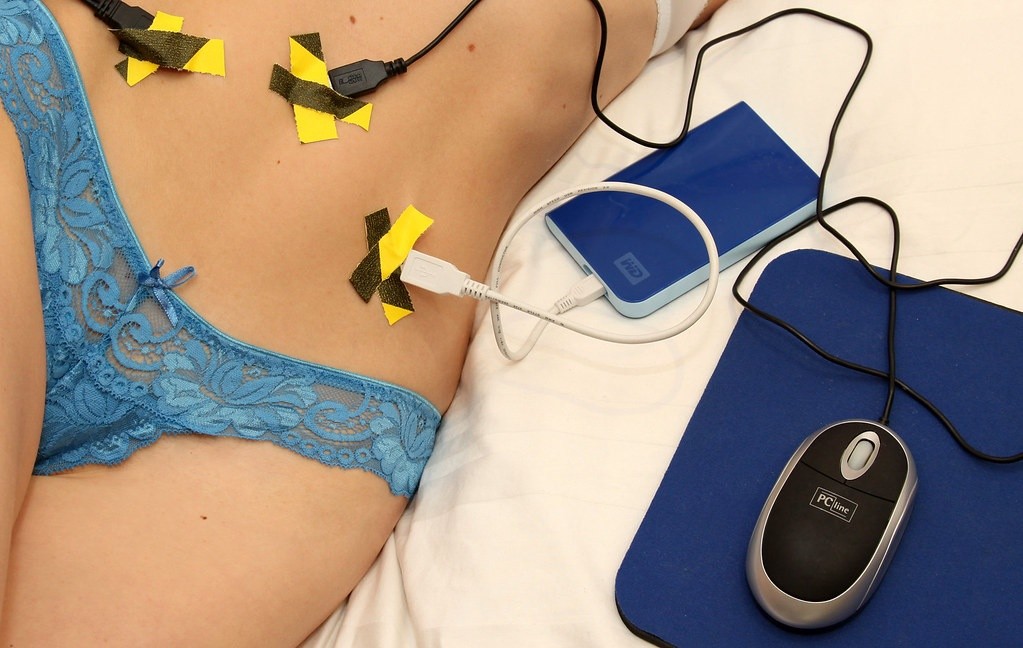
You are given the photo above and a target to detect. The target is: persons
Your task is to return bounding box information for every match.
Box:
[0,0,720,648]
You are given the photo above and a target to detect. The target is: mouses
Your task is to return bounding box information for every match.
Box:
[744,418,920,628]
